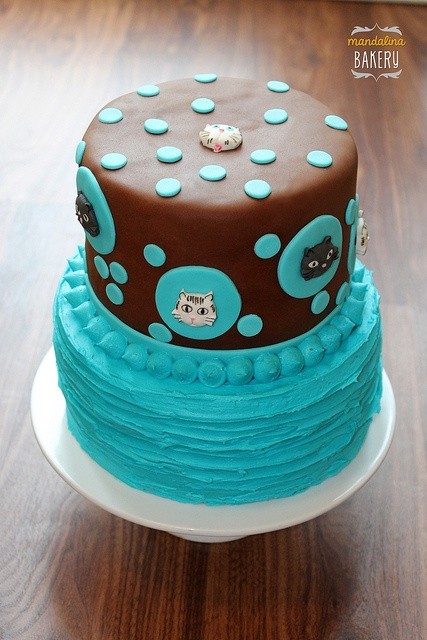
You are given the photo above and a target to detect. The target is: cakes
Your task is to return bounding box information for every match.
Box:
[50,72,382,507]
